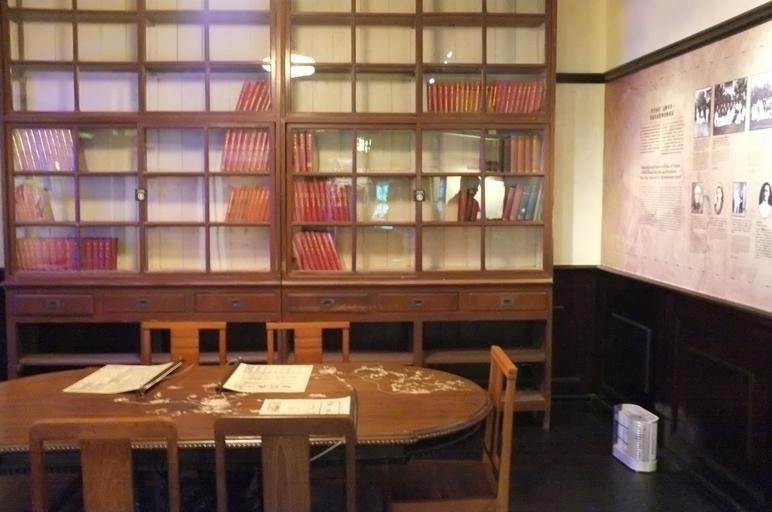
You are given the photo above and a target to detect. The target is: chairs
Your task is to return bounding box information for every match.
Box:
[213,415,358,511]
[140,322,226,368]
[381,345,518,509]
[32,415,179,510]
[266,322,349,364]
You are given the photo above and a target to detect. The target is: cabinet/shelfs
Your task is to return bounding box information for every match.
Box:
[2,1,277,377]
[280,2,557,435]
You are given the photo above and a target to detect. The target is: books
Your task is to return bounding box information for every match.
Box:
[427,81,548,221]
[12,126,118,270]
[221,79,352,271]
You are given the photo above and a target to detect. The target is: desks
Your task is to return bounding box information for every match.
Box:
[0,359,495,512]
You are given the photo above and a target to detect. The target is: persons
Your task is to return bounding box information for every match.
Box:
[758,184,772,217]
[714,186,723,214]
[695,96,772,128]
[691,184,703,213]
